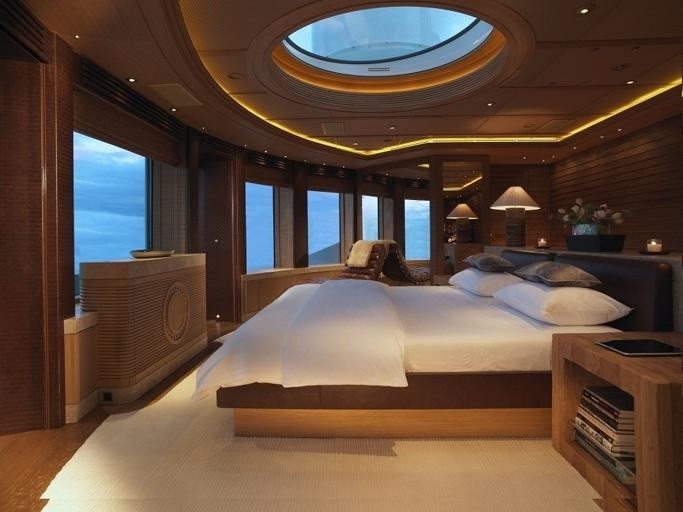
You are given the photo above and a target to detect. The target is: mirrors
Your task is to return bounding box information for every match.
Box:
[441,161,484,274]
[361,162,431,261]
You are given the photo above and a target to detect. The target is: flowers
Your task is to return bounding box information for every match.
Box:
[559,197,625,225]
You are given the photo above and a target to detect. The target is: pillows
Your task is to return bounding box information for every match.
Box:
[462,252,515,271]
[514,260,603,287]
[492,280,634,326]
[448,267,525,296]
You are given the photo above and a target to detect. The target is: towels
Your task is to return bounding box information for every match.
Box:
[346,239,379,268]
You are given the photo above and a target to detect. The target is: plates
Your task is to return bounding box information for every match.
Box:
[639,249,672,255]
[129,250,176,258]
[535,246,551,249]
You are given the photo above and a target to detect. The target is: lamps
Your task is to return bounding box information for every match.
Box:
[490,186,542,246]
[446,203,480,243]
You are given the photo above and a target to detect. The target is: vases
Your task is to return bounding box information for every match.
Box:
[564,223,626,253]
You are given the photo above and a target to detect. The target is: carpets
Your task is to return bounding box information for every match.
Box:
[39,332,604,511]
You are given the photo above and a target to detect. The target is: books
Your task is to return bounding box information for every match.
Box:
[568,384,636,488]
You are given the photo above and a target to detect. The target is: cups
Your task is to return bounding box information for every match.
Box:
[537,238,547,247]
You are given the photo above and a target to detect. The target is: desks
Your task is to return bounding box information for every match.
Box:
[433,274,453,286]
[79,253,209,405]
[551,332,683,512]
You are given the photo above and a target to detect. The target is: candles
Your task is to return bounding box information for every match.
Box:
[538,237,547,247]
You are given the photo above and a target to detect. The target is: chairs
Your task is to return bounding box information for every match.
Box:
[297,239,386,286]
[382,240,431,286]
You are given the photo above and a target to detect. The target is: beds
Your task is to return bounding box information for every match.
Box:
[216,249,674,440]
